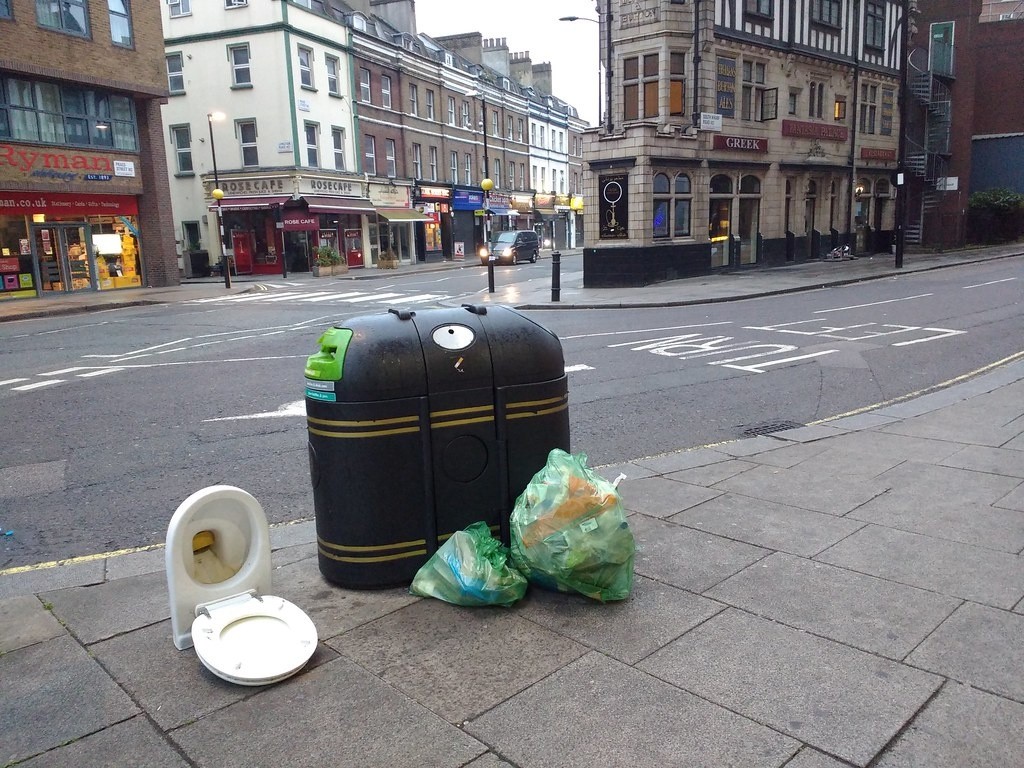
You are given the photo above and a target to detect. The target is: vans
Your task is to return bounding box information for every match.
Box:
[479,230,539,266]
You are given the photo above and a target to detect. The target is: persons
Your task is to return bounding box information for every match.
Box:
[709,208,721,238]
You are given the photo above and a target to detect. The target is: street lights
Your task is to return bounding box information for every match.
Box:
[465,91,496,294]
[207,112,236,289]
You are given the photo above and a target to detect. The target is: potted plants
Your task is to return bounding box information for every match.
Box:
[377,248,399,270]
[965,186,1024,244]
[311,246,348,276]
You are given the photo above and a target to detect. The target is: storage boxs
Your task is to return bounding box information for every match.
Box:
[96,235,141,290]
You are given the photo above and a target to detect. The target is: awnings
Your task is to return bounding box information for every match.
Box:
[208,196,292,212]
[489,209,520,216]
[375,208,434,222]
[303,195,376,215]
[535,209,559,222]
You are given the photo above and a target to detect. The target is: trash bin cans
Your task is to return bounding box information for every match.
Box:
[305,303,572,590]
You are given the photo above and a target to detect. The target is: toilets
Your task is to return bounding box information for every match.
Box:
[165,485,319,686]
